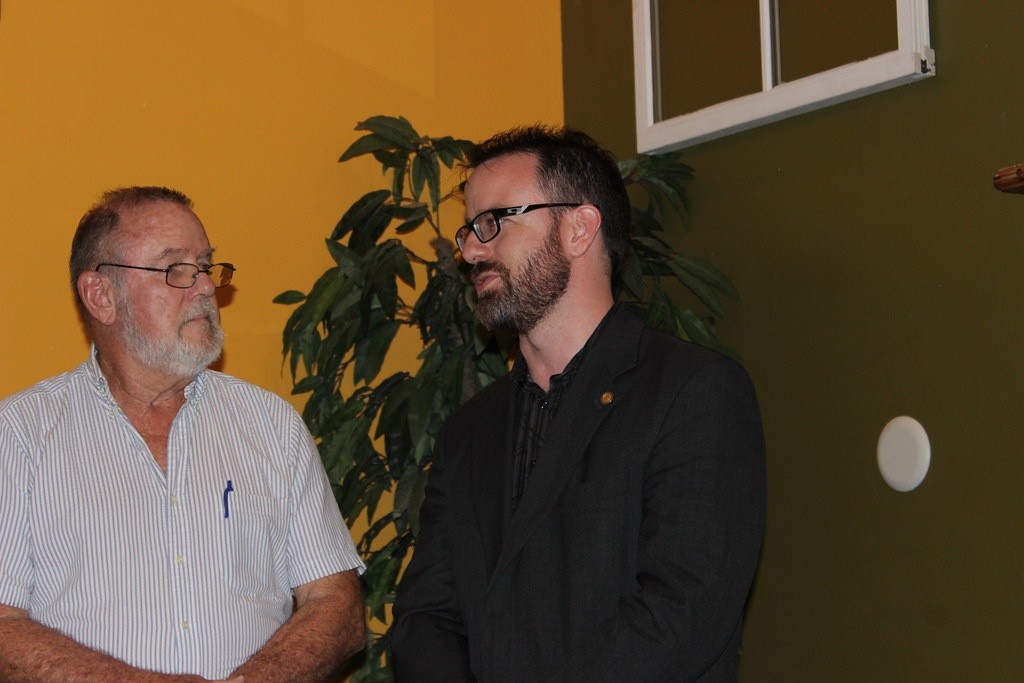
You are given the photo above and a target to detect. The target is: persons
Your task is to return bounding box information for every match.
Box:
[392,121,765,683]
[0,185,369,683]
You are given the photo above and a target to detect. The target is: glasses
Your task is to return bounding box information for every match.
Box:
[455,202,600,253]
[96,263,234,288]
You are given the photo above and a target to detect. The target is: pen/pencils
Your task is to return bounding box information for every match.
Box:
[223,480,234,518]
